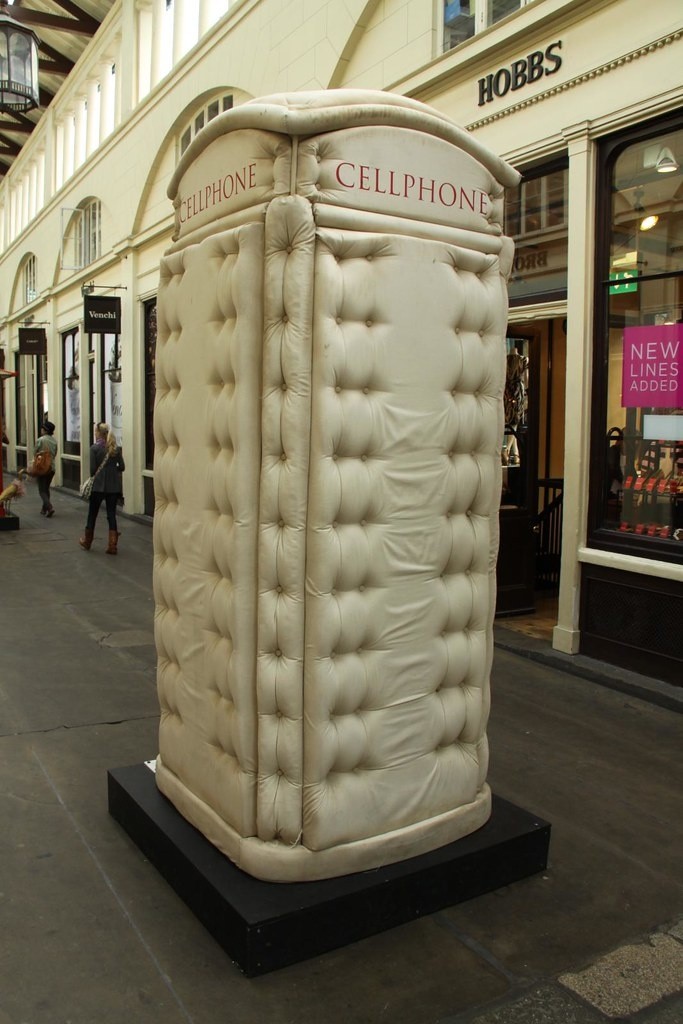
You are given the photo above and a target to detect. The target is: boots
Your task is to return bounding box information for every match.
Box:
[47,504,55,517]
[40,505,47,514]
[80,526,94,550]
[106,530,121,554]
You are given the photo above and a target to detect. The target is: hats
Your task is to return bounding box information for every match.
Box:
[41,422,55,432]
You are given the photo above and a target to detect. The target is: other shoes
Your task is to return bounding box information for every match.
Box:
[513,455,520,464]
[502,448,511,466]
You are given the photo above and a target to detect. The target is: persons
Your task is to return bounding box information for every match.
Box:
[79,421,126,555]
[34,421,58,517]
[1,431,9,445]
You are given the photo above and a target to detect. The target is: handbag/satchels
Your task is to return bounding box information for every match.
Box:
[32,436,50,477]
[81,478,94,501]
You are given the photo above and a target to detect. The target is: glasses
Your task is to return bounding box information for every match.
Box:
[97,422,101,431]
